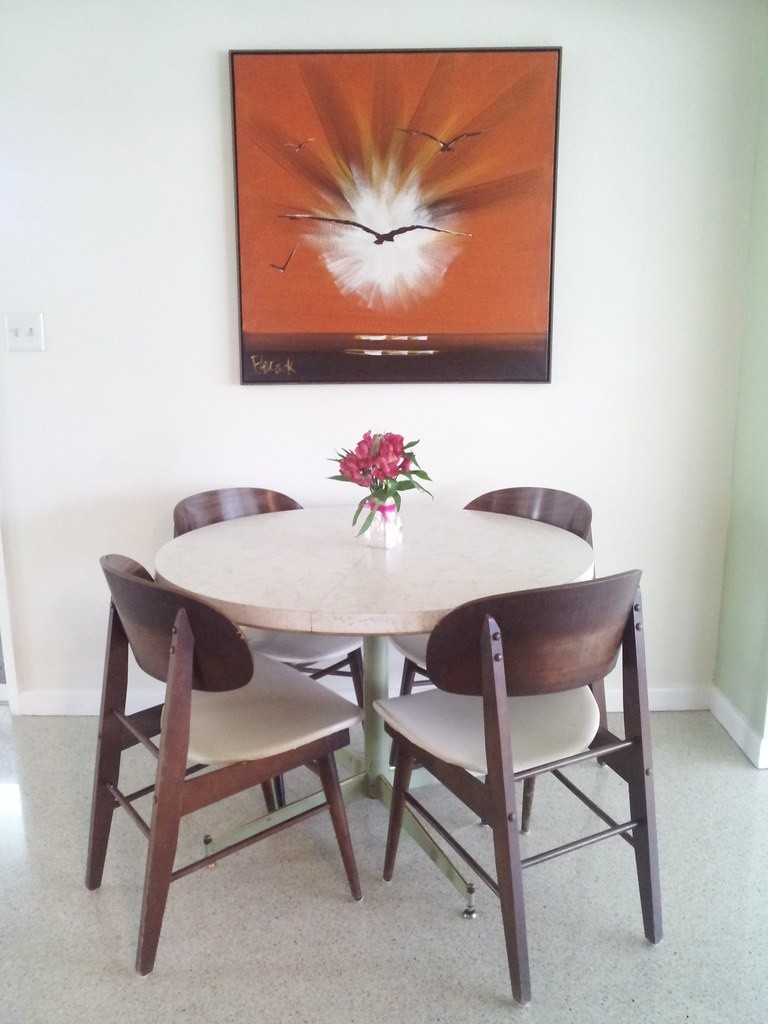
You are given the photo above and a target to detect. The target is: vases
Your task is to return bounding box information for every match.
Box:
[359,491,408,553]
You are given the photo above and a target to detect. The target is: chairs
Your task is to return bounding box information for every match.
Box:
[172,487,365,812]
[384,485,593,835]
[84,554,364,975]
[371,568,663,1004]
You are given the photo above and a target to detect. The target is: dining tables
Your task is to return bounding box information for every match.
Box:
[155,500,600,921]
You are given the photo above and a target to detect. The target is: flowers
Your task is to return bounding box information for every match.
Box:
[322,427,434,537]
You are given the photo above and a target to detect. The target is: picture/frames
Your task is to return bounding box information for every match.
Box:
[226,47,563,385]
